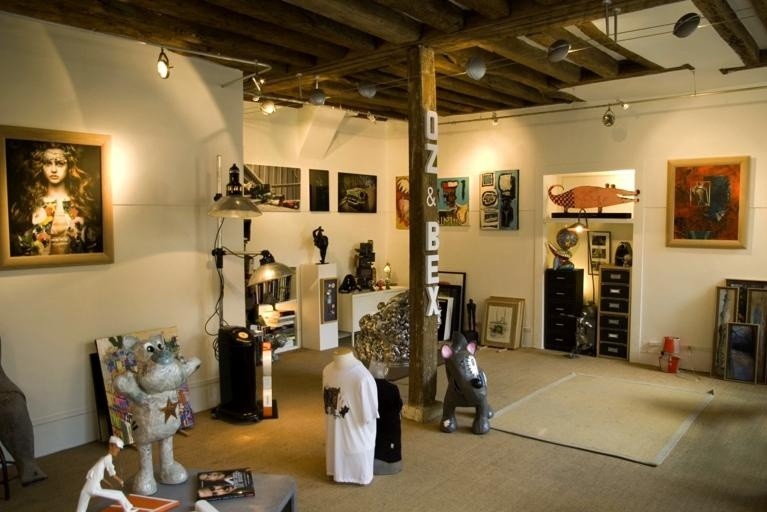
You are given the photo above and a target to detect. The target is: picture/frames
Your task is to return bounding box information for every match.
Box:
[479,295,525,351]
[587,231,611,276]
[1,125,114,269]
[435,271,466,341]
[711,279,767,387]
[666,155,750,249]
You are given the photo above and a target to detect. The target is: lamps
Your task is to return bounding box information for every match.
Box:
[156,47,173,77]
[566,209,596,305]
[208,155,265,327]
[218,249,294,289]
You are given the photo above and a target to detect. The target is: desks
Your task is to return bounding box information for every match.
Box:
[89,465,297,512]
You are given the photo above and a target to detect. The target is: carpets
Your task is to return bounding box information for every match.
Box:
[489,372,714,469]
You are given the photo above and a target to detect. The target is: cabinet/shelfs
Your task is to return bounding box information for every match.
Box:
[337,285,408,347]
[245,264,302,355]
[596,264,633,361]
[543,268,584,353]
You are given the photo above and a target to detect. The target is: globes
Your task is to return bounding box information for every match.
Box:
[555,229,579,257]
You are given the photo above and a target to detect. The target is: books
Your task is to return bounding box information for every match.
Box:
[195,466,256,501]
[101,493,180,512]
[253,273,296,350]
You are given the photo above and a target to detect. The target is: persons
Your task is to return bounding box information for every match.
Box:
[197,482,239,498]
[560,311,595,358]
[9,141,103,256]
[198,471,234,485]
[76,435,141,512]
